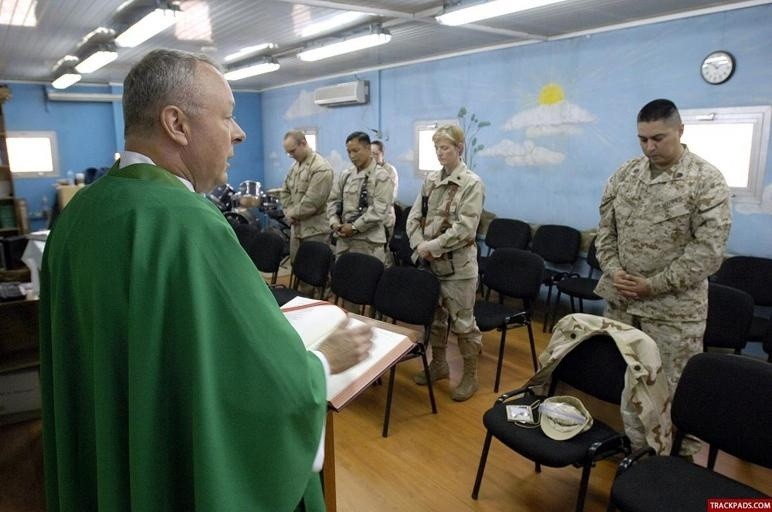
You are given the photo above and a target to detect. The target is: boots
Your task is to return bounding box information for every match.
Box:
[414,346,450,385]
[451,351,479,402]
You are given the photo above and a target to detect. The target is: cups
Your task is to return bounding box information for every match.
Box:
[58,167,97,188]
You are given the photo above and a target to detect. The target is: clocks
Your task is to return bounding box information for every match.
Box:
[700,50,736,85]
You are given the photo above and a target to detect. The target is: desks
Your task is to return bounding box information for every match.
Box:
[0,269,40,373]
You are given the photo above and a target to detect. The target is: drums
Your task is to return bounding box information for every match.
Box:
[209,183,234,209]
[223,208,254,225]
[263,195,278,207]
[238,180,262,207]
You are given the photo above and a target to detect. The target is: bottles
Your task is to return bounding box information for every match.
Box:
[14,197,33,234]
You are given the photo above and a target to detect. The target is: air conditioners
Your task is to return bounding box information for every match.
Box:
[313,80,369,107]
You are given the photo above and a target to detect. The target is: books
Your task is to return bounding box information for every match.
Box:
[278,294,418,413]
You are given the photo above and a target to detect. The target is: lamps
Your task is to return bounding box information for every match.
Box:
[296,21,391,62]
[431,0,566,26]
[51,0,184,90]
[223,54,280,81]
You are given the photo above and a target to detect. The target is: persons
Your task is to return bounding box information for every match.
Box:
[325,130,393,322]
[370,140,399,253]
[405,123,487,401]
[592,97,732,462]
[36,43,374,512]
[279,129,334,301]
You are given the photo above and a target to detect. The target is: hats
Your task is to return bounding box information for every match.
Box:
[539,395,594,441]
